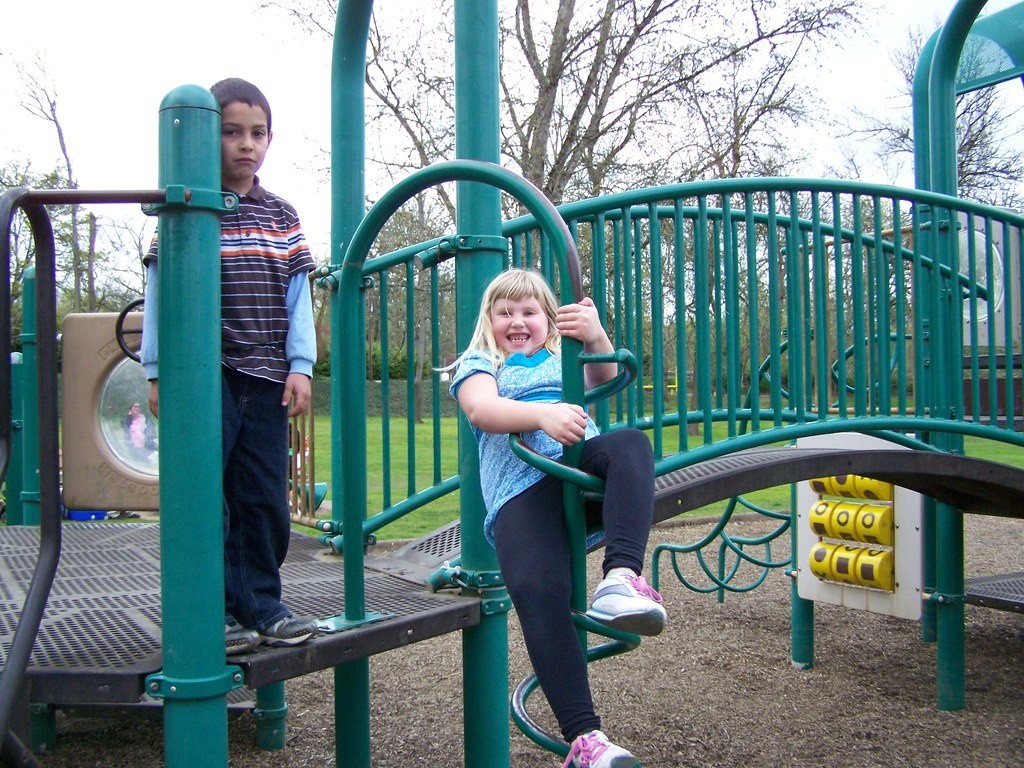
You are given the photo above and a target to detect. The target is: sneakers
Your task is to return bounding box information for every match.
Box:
[560,730,637,768]
[585,574,667,636]
[258,613,317,647]
[225,621,259,655]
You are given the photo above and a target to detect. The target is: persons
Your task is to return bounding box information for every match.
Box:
[447,268,667,768]
[141,77,318,654]
[121,401,155,461]
[288,423,300,490]
